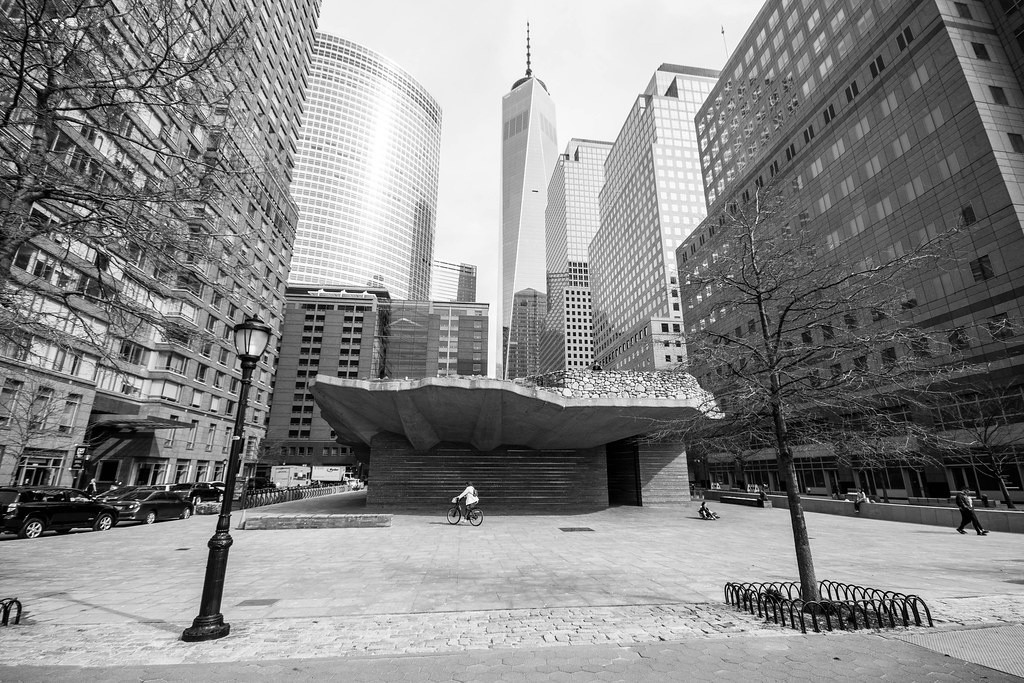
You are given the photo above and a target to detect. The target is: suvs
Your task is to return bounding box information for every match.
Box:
[0,485,121,539]
[150,484,178,491]
[233,476,276,501]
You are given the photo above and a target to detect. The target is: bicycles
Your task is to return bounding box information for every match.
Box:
[447,496,484,526]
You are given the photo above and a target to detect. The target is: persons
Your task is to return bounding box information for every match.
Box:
[757,489,768,507]
[955,488,990,535]
[854,487,866,512]
[86,479,96,494]
[699,502,720,520]
[831,480,838,500]
[110,479,118,490]
[457,481,479,523]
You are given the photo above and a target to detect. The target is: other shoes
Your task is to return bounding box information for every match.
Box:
[978,531,986,535]
[956,528,967,534]
[983,530,990,532]
[855,510,860,513]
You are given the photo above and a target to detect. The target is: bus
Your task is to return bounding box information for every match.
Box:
[346,479,364,490]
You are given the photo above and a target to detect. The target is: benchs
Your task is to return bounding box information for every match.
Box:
[719,496,773,508]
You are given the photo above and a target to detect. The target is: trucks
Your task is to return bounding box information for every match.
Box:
[311,465,356,488]
[270,465,311,491]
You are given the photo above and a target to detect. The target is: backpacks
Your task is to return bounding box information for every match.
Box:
[468,486,478,497]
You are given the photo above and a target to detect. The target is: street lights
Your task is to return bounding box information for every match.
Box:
[181,313,272,642]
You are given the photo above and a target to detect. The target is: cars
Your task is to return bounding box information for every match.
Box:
[107,489,194,524]
[209,481,226,491]
[170,482,224,505]
[93,485,151,503]
